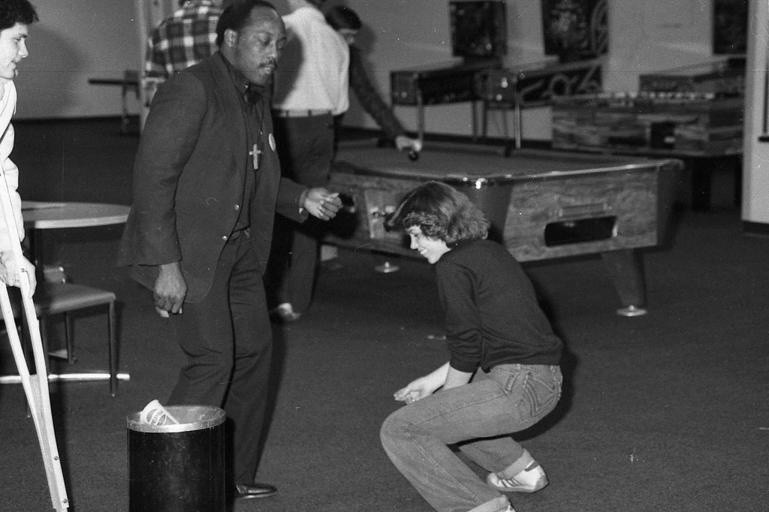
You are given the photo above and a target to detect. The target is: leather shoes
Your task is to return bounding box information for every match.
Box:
[236,480,276,499]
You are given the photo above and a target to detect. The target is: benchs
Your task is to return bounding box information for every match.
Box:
[0,282,118,396]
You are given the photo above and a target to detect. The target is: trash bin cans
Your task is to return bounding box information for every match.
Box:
[125,404,228,512]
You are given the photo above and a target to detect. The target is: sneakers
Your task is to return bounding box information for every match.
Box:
[486,457,548,494]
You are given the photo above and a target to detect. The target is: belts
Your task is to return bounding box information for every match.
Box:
[274,108,330,119]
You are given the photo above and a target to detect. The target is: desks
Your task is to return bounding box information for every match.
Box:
[19,201,137,281]
[321,138,685,318]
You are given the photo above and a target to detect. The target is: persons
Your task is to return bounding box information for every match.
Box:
[322,7,419,162]
[143,0,227,112]
[268,1,349,324]
[380,180,565,512]
[112,0,344,501]
[0,2,39,304]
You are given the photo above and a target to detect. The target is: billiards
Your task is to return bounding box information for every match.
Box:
[409,150,418,160]
